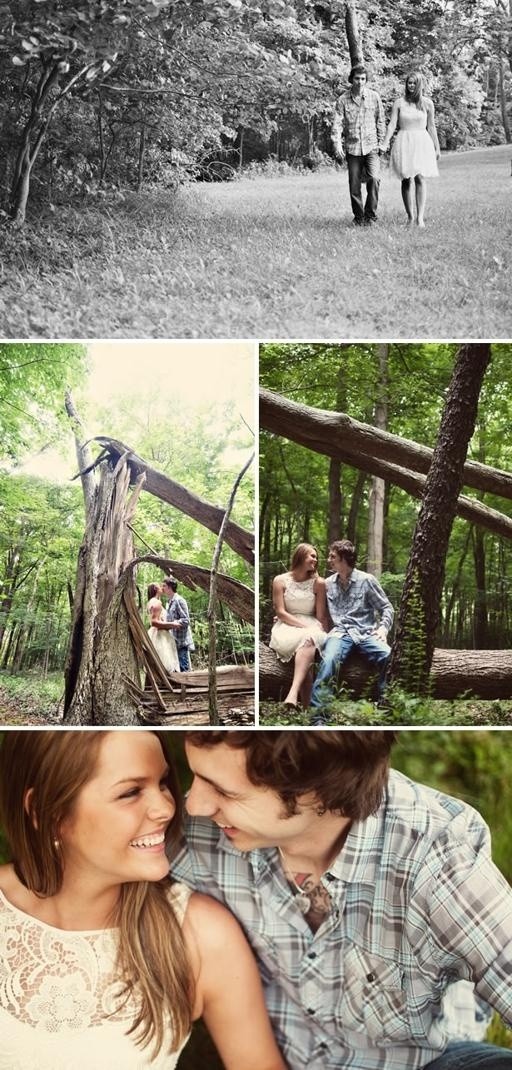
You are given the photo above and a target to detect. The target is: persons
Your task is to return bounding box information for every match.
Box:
[330,65,387,228]
[147,582,183,674]
[164,730,512,1070]
[309,540,394,726]
[382,73,441,227]
[269,542,330,710]
[161,578,195,672]
[0,731,287,1070]
